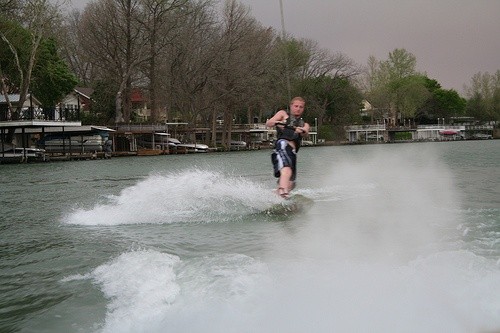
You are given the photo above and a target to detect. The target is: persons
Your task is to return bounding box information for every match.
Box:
[265,96,310,201]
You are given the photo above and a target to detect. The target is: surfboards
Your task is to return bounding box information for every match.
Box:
[259,192,314,216]
